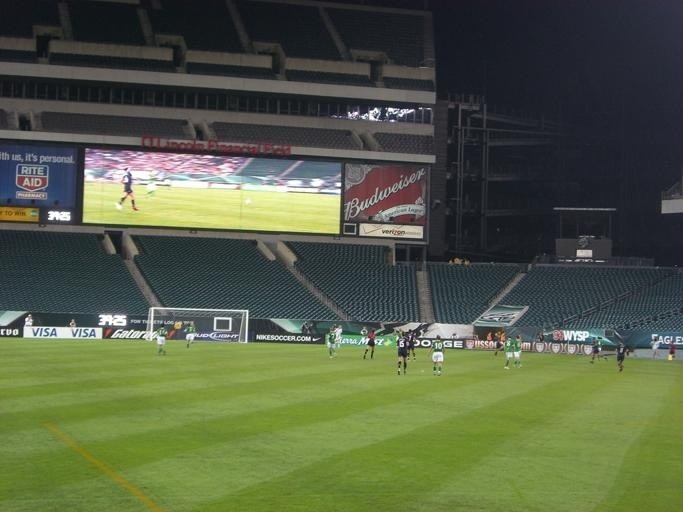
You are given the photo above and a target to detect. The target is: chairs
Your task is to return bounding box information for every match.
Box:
[0,230,683,333]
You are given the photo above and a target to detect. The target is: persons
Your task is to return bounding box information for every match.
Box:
[24,314,34,326]
[115,167,137,211]
[648,334,662,361]
[155,323,167,354]
[588,336,608,363]
[363,328,375,360]
[332,324,342,348]
[326,328,337,359]
[427,333,444,378]
[667,340,677,359]
[69,318,76,328]
[394,329,408,375]
[614,342,626,372]
[181,321,196,349]
[405,328,416,361]
[483,329,545,369]
